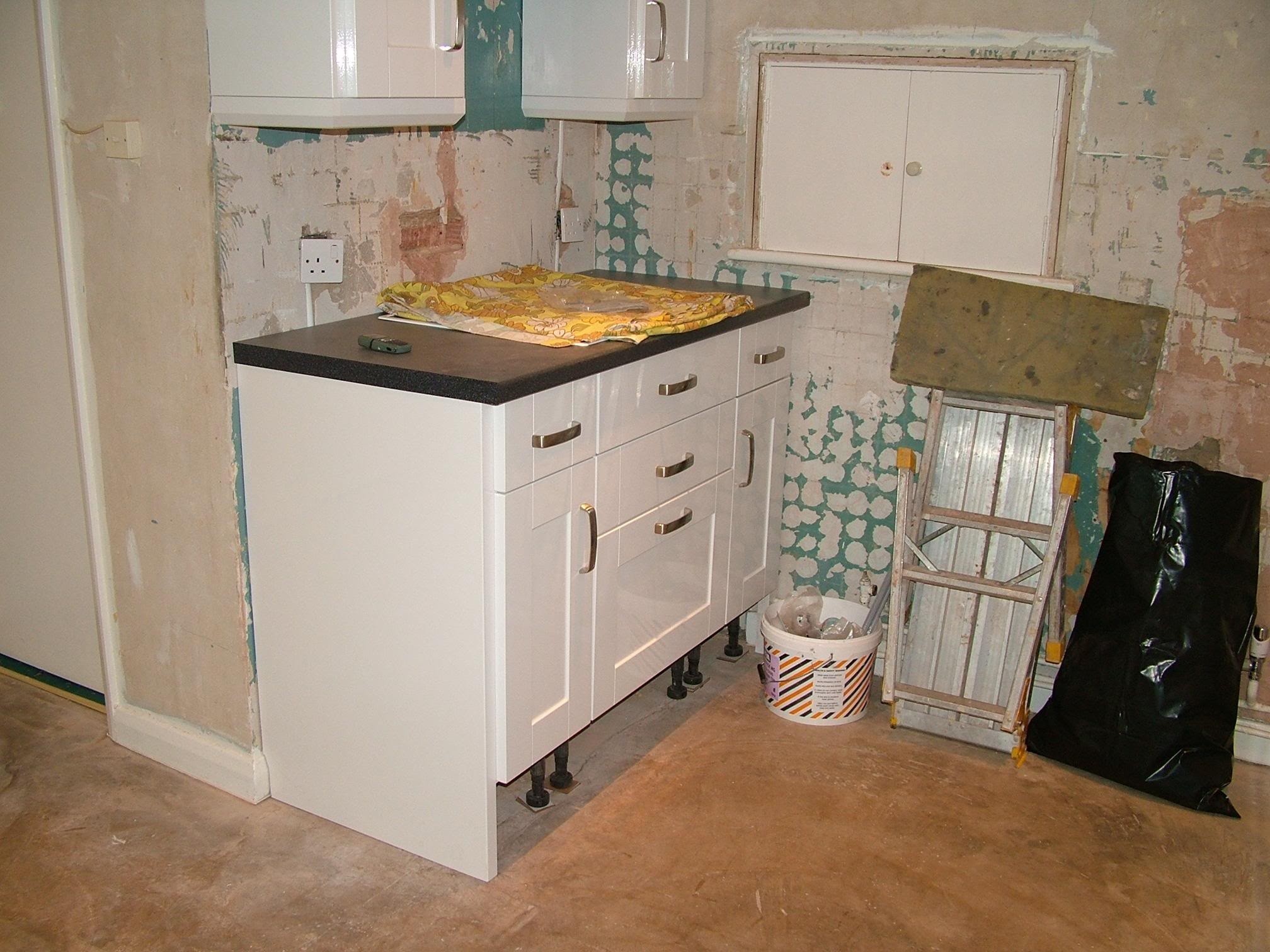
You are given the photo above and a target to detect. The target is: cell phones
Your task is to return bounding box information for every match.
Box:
[358,333,413,355]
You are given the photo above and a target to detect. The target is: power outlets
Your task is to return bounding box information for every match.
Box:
[556,207,584,243]
[299,238,343,283]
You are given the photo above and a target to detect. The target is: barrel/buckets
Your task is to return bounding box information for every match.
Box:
[759,595,884,726]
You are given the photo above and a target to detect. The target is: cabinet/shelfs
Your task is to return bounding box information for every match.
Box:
[204,0,466,129]
[522,0,706,121]
[237,311,792,882]
